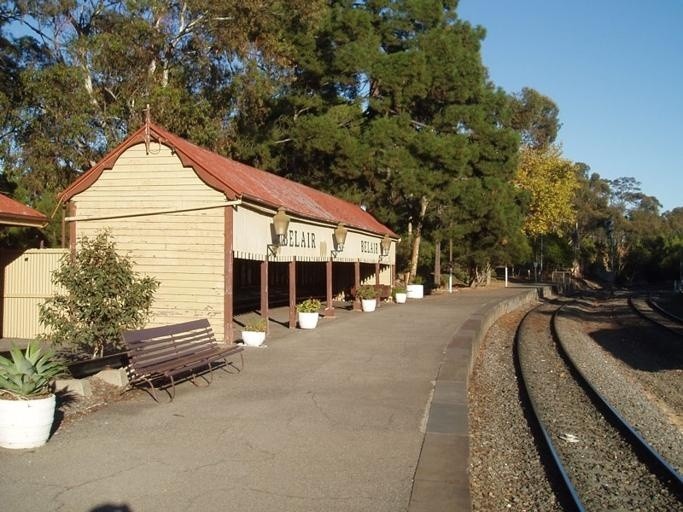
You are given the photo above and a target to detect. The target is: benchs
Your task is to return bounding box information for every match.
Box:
[121,317,246,404]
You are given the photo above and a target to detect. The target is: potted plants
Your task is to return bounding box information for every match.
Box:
[35,224,161,379]
[295,298,321,329]
[393,287,407,303]
[240,316,268,347]
[356,284,378,313]
[0,337,75,451]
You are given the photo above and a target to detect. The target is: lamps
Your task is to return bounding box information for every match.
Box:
[331,221,347,257]
[378,231,392,262]
[267,205,290,257]
